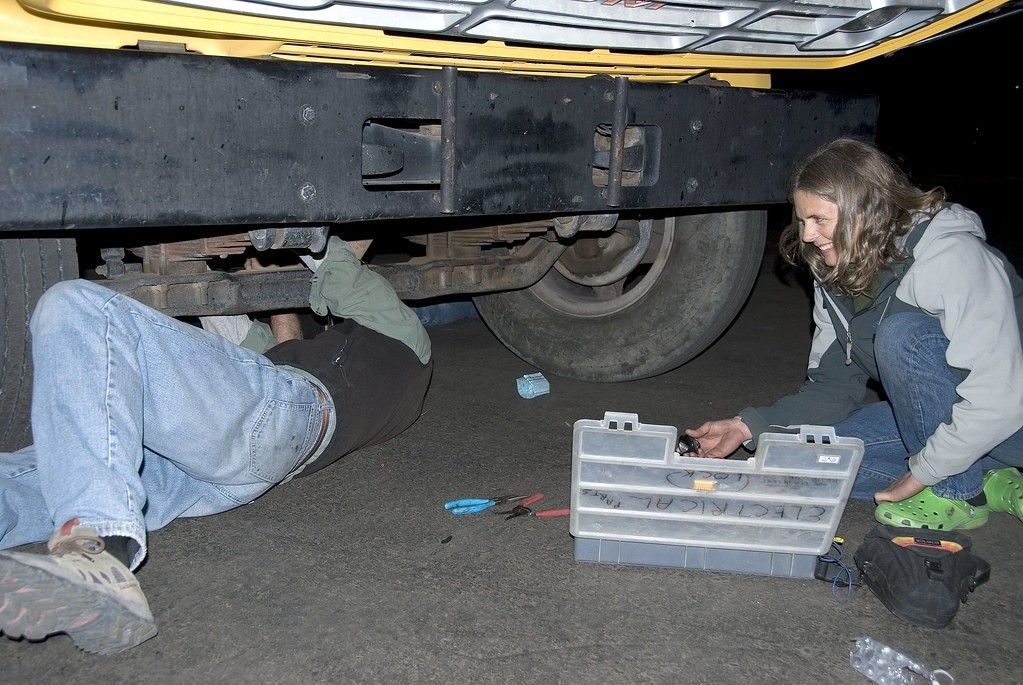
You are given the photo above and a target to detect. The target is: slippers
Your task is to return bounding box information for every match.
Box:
[982,467,1023,522]
[875,487,988,531]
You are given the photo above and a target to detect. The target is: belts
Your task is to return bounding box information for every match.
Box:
[283,380,330,479]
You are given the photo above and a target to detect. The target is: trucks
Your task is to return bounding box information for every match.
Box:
[0,0,1021,454]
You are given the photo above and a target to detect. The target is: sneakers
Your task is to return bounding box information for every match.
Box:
[0,517,158,656]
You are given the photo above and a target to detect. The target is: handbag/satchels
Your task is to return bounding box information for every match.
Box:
[815,524,991,629]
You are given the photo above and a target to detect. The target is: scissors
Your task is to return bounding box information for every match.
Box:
[494,492,570,522]
[444,493,527,516]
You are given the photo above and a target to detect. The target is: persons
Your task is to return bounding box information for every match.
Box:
[676,138,1023,531]
[0,236,433,656]
